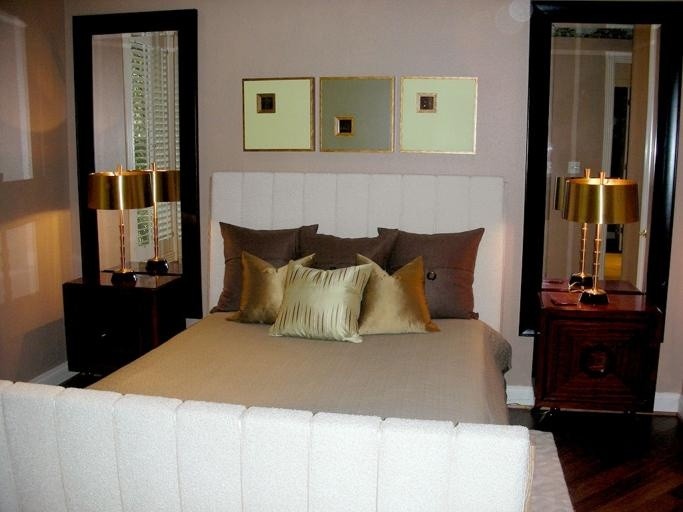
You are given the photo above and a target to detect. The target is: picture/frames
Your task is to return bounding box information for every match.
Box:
[241,78,316,152]
[400,76,477,155]
[319,76,395,153]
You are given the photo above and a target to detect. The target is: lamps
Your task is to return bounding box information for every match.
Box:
[555,169,593,288]
[141,163,181,271]
[88,164,162,282]
[562,172,638,305]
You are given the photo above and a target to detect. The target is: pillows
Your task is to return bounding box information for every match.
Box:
[299,225,401,274]
[354,252,442,336]
[376,226,485,319]
[210,221,319,313]
[267,259,373,343]
[227,251,318,324]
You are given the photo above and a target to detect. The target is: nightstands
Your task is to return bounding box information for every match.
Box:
[60,274,184,374]
[536,290,664,414]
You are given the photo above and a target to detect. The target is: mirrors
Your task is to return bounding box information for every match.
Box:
[522,0,682,297]
[71,8,200,278]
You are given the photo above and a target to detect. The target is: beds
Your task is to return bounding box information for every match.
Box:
[0,170,536,511]
